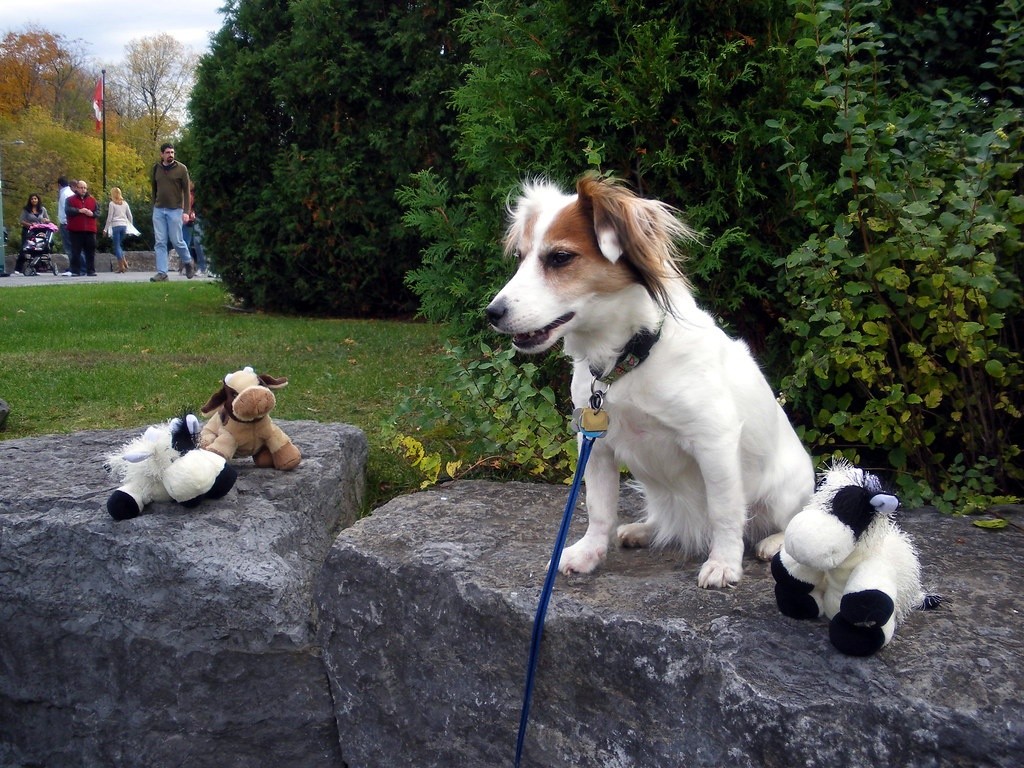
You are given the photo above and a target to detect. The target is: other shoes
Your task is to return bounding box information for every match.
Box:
[186,259,194,279]
[150,272,169,281]
[71,272,80,276]
[87,273,97,276]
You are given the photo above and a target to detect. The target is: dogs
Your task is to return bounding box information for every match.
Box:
[483,174,818,592]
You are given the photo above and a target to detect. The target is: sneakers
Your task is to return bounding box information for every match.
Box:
[208,271,218,277]
[61,270,73,276]
[195,269,206,276]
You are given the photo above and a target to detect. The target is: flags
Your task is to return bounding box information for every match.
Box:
[93,76,103,134]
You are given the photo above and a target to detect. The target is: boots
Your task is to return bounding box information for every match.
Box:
[115,256,129,273]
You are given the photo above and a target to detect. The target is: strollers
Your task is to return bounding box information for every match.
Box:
[21,222,58,276]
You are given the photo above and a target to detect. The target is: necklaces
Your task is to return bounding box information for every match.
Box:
[34,210,35,211]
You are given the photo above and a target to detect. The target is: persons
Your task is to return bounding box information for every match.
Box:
[14,193,50,275]
[150,143,208,282]
[102,187,132,274]
[58,176,101,277]
